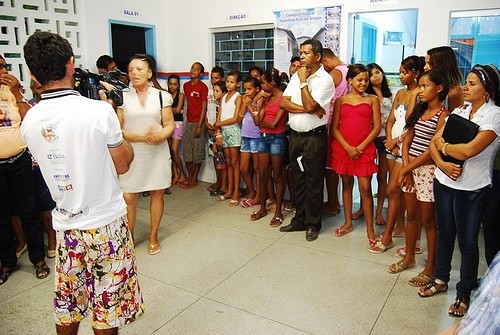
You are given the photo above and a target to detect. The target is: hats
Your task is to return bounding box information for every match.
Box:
[473,63,499,95]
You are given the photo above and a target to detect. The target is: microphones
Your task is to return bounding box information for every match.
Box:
[110,70,127,77]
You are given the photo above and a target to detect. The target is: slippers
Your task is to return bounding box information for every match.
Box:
[229,199,240,207]
[333,225,353,236]
[250,211,267,221]
[407,273,435,286]
[265,198,340,215]
[368,239,394,253]
[209,188,226,196]
[387,260,408,274]
[216,194,229,202]
[148,233,161,255]
[270,214,284,227]
[367,235,380,247]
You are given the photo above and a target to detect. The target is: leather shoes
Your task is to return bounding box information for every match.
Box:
[306,227,318,242]
[280,223,307,232]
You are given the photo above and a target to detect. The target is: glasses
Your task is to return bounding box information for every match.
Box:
[0,63,12,71]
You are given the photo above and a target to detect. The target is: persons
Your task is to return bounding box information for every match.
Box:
[115,53,175,255]
[279,39,336,243]
[387,68,450,289]
[0,29,500,334]
[330,62,382,248]
[180,62,208,189]
[417,63,500,319]
[19,29,146,334]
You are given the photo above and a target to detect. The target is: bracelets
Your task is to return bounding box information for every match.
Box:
[252,112,259,116]
[239,112,245,118]
[440,141,449,157]
[397,135,404,143]
[252,104,258,108]
[215,132,222,139]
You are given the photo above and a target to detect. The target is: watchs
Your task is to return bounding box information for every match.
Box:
[16,98,27,103]
[299,81,309,90]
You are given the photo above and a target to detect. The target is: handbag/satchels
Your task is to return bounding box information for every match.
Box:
[198,147,217,183]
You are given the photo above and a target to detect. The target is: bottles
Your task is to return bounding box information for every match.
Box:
[253,104,258,116]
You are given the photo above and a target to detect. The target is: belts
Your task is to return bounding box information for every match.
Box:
[290,127,327,137]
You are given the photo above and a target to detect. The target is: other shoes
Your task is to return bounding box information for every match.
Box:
[47,247,56,258]
[380,229,406,238]
[394,244,423,258]
[241,199,253,208]
[16,244,27,259]
[352,210,363,220]
[375,216,387,225]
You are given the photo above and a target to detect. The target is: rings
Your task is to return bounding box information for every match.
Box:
[8,80,12,83]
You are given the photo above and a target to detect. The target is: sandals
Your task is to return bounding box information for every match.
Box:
[33,261,51,279]
[0,260,18,285]
[417,279,448,297]
[448,297,469,317]
